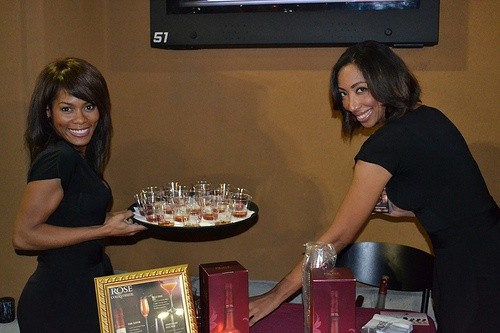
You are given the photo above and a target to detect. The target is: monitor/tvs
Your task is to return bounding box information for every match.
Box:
[150,0,441,50]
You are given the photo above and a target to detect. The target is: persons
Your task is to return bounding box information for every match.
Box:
[249,40,500,333]
[12,57,148,333]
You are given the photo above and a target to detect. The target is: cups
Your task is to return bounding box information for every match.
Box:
[0,296,16,323]
[133,181,252,227]
[154,317,166,333]
[373,187,387,211]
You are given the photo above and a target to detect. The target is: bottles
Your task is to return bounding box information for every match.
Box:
[113,307,126,333]
[220,283,241,333]
[330,290,339,333]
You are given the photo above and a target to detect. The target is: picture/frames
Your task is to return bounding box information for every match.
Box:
[93,263,198,333]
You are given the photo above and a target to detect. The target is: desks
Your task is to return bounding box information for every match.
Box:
[249,302,437,333]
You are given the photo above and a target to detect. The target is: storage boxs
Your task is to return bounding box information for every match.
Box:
[199,260,249,333]
[310,268,357,333]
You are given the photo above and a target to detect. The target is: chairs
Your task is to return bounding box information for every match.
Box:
[335,241,434,316]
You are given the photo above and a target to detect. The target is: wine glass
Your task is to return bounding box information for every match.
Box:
[161,280,178,312]
[140,296,151,333]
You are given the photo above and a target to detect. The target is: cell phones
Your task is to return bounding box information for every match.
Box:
[372,187,390,213]
